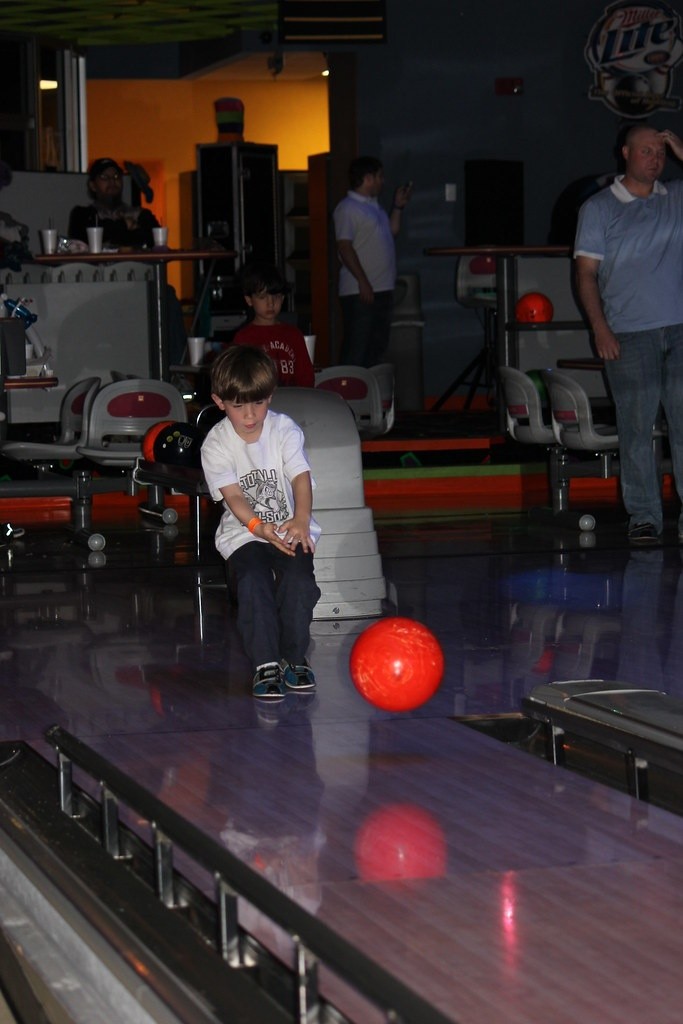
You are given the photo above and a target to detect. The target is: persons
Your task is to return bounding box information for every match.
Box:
[570,125,683,546]
[331,155,413,369]
[608,549,683,705]
[232,260,315,388]
[0,161,29,268]
[223,689,325,959]
[67,158,191,379]
[201,347,322,697]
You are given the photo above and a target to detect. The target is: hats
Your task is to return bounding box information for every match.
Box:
[89,157,124,177]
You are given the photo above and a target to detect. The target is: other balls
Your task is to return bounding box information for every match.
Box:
[354,801,450,882]
[516,293,554,323]
[142,420,200,467]
[348,615,443,713]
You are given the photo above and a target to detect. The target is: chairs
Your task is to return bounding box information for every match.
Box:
[0,364,398,569]
[500,367,660,516]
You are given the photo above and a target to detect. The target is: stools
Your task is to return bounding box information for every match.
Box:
[556,357,615,407]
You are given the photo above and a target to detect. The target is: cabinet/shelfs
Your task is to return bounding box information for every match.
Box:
[281,171,312,312]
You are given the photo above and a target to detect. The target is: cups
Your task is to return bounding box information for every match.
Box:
[187,337,205,367]
[86,226,104,254]
[152,227,168,251]
[303,335,316,365]
[25,344,33,360]
[40,229,58,255]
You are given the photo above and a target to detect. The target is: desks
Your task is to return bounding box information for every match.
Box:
[3,377,59,388]
[422,247,592,437]
[35,251,240,382]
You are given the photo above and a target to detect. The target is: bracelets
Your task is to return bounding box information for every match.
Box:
[246,516,264,533]
[391,203,405,210]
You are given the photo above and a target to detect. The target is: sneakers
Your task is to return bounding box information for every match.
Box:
[253,697,287,723]
[629,523,658,546]
[252,665,286,697]
[280,656,316,688]
[284,689,316,708]
